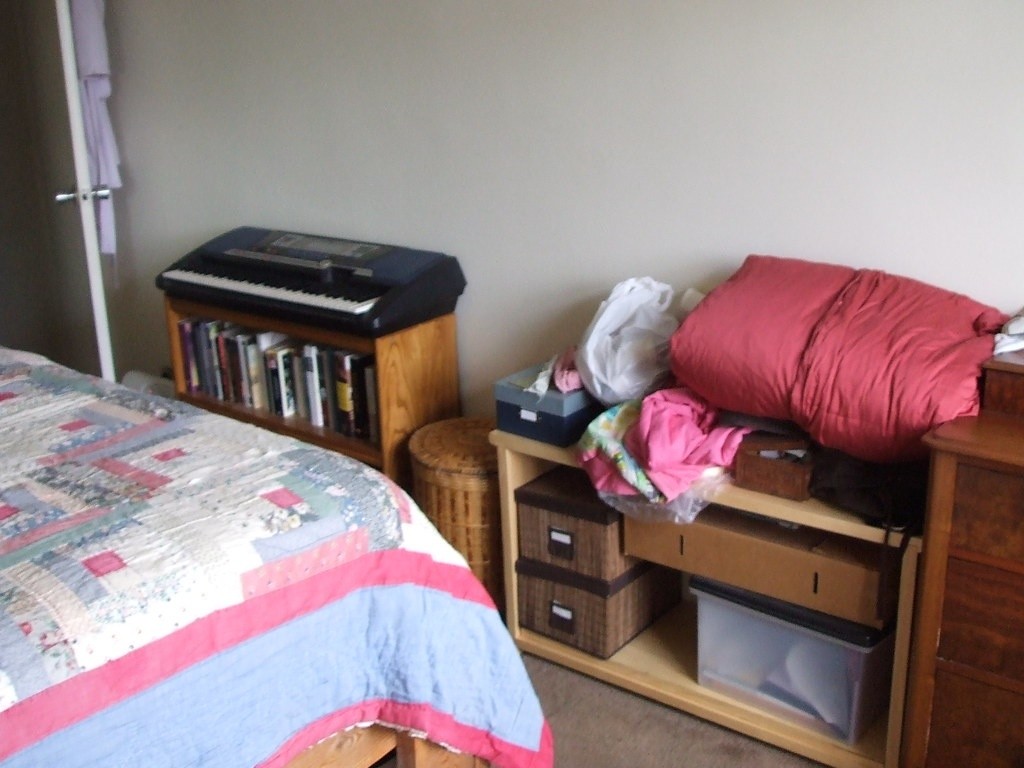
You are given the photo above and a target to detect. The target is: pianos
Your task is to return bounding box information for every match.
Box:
[156,224,467,338]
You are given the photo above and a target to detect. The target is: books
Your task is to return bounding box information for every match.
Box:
[256,332,288,413]
[176,318,382,446]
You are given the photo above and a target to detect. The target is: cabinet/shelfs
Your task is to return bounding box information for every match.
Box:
[487,432,924,768]
[163,294,463,494]
[902,350,1024,768]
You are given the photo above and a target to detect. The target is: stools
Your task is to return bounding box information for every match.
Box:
[412,414,509,620]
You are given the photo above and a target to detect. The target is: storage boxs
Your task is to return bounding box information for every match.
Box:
[735,421,836,502]
[686,576,896,746]
[494,362,600,450]
[513,463,644,581]
[622,504,900,626]
[513,556,682,660]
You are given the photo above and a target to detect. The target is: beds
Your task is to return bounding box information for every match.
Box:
[0,348,558,768]
